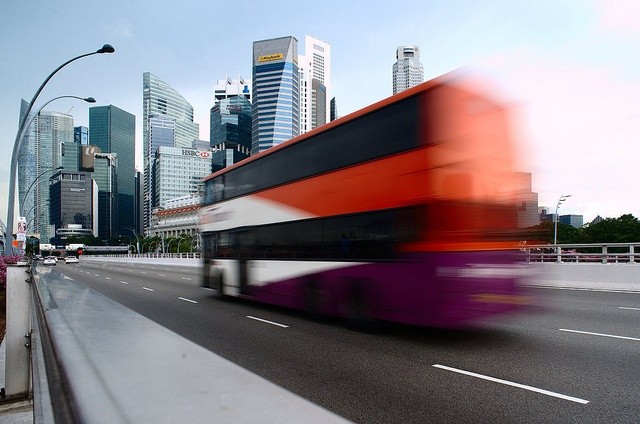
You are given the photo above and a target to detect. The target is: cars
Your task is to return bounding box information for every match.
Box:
[44,256,56,266]
[66,255,78,263]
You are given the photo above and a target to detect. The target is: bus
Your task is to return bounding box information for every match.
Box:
[197,66,536,327]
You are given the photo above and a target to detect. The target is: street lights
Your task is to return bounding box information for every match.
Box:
[22,164,64,215]
[554,192,572,252]
[26,197,50,230]
[5,43,115,255]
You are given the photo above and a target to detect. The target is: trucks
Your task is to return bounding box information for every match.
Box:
[66,243,84,250]
[39,243,55,250]
